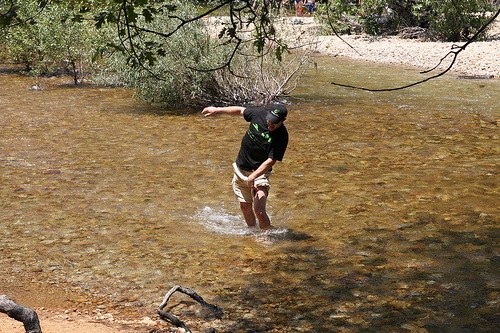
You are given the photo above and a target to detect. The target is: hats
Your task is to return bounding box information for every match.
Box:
[265,104,287,124]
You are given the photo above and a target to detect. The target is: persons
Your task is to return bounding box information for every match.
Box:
[202,104,289,229]
[294,0,315,17]
